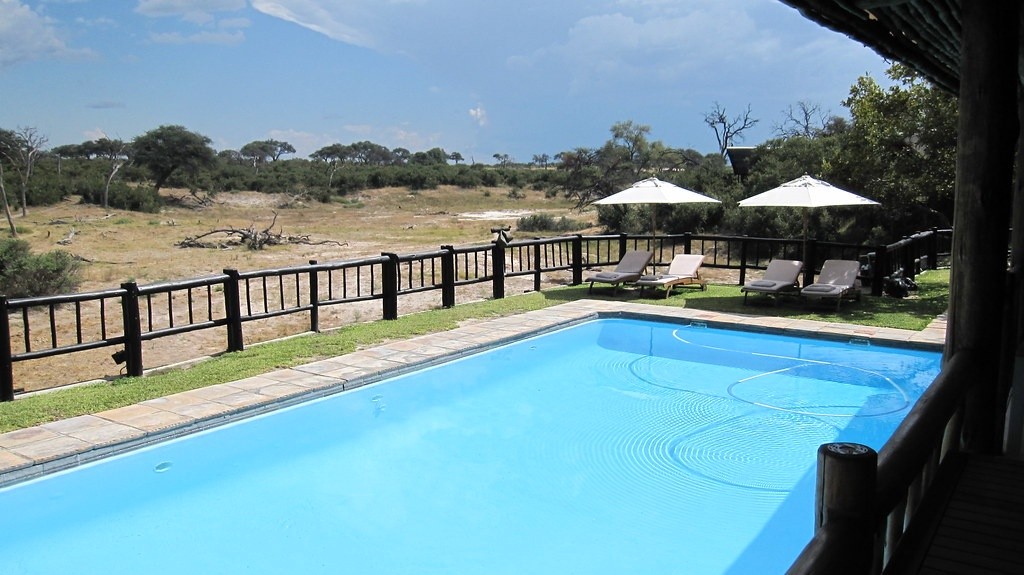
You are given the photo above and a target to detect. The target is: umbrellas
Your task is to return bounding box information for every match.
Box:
[591,174,723,275]
[736,172,882,289]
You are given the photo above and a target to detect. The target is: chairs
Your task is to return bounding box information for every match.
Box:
[741,258,803,307]
[585,250,655,297]
[801,260,860,312]
[636,254,707,300]
